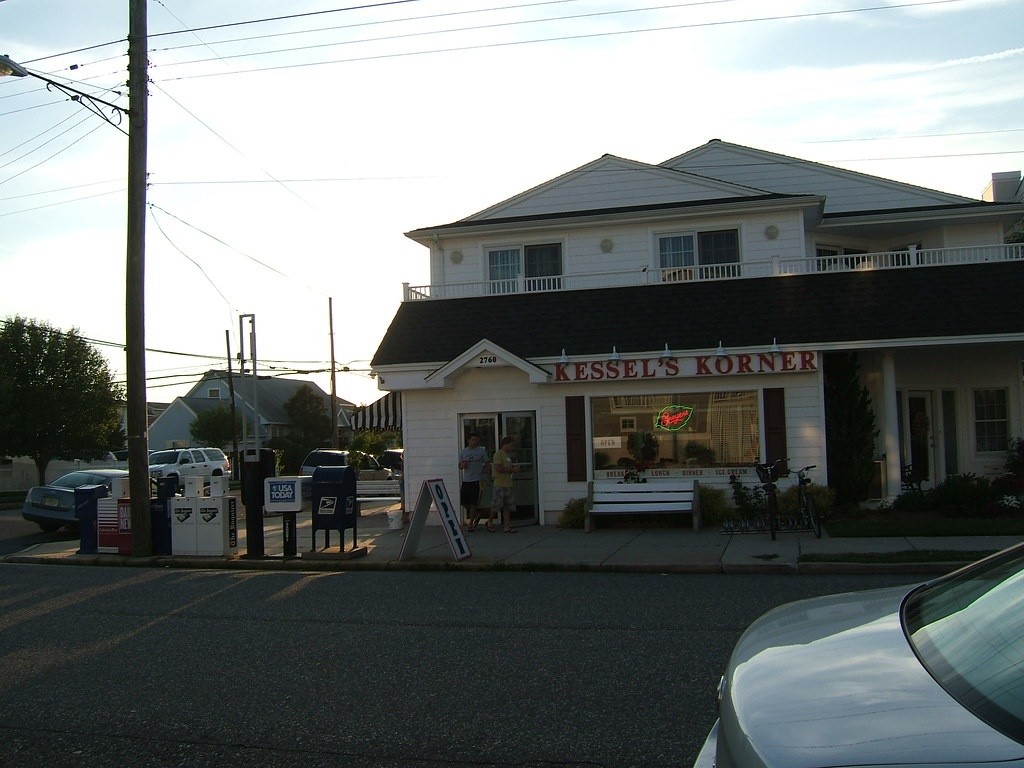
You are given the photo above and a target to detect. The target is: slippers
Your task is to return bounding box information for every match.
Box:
[486,522,496,532]
[473,514,483,527]
[468,526,474,532]
[504,529,518,533]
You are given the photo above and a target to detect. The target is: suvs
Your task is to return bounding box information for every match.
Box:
[299,450,392,481]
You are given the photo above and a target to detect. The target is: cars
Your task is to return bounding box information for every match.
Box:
[114,450,157,467]
[378,449,404,477]
[692,538,1023,768]
[21,466,129,531]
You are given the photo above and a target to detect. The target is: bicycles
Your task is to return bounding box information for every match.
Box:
[750,459,792,541]
[782,466,823,540]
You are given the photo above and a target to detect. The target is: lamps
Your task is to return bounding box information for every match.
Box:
[609,346,620,361]
[662,342,673,357]
[559,349,569,363]
[716,340,728,355]
[770,338,782,352]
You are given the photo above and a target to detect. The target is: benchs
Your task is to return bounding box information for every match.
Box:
[582,479,702,533]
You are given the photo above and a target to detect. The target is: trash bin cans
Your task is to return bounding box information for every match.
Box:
[312,466,358,551]
[75,483,106,554]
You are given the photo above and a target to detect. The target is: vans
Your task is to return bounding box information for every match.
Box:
[148,446,232,488]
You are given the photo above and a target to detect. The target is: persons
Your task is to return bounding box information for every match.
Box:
[485,436,521,533]
[458,433,491,533]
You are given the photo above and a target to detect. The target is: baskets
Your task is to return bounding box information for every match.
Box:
[756,464,779,483]
